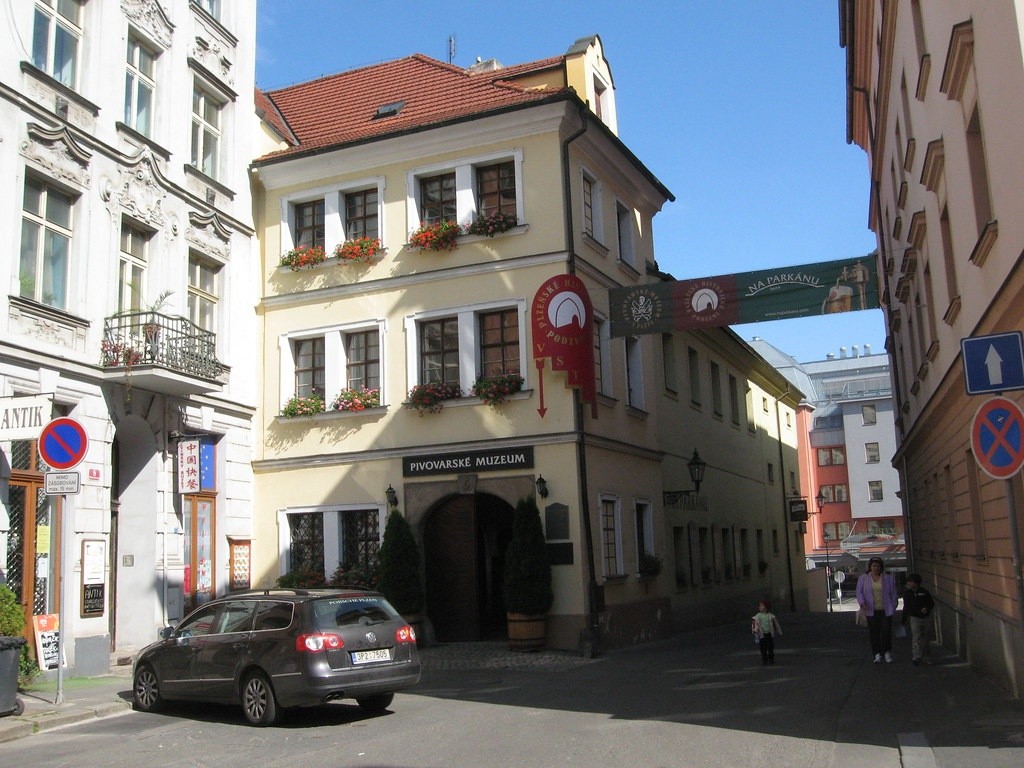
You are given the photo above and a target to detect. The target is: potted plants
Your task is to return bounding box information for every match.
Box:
[109,281,175,343]
[379,510,425,645]
[640,553,664,577]
[503,494,555,650]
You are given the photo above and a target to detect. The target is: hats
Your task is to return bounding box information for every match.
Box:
[907,574,921,585]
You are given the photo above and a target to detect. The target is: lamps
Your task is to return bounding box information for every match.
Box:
[662,447,706,506]
[536,473,548,500]
[386,484,398,508]
[806,490,826,518]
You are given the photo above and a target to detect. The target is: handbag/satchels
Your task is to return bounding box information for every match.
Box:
[895,623,908,638]
[856,609,868,626]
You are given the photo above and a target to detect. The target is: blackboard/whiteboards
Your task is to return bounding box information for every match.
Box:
[80,539,107,618]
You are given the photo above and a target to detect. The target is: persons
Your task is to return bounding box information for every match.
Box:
[903,573,934,664]
[751,601,782,664]
[856,557,897,663]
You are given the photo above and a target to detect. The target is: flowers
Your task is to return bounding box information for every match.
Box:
[99,340,143,403]
[330,561,379,589]
[406,381,465,417]
[275,560,330,589]
[281,387,325,419]
[406,217,463,255]
[468,368,525,406]
[280,243,329,272]
[463,210,518,238]
[333,236,389,266]
[334,384,381,412]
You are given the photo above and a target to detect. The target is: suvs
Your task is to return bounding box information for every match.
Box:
[131,587,421,728]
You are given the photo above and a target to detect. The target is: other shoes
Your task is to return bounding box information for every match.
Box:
[873,655,882,664]
[911,657,920,665]
[923,658,933,665]
[884,652,893,663]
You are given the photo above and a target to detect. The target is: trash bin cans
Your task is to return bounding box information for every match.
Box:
[0,636,28,717]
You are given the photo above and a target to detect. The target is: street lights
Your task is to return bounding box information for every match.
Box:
[822,529,833,611]
